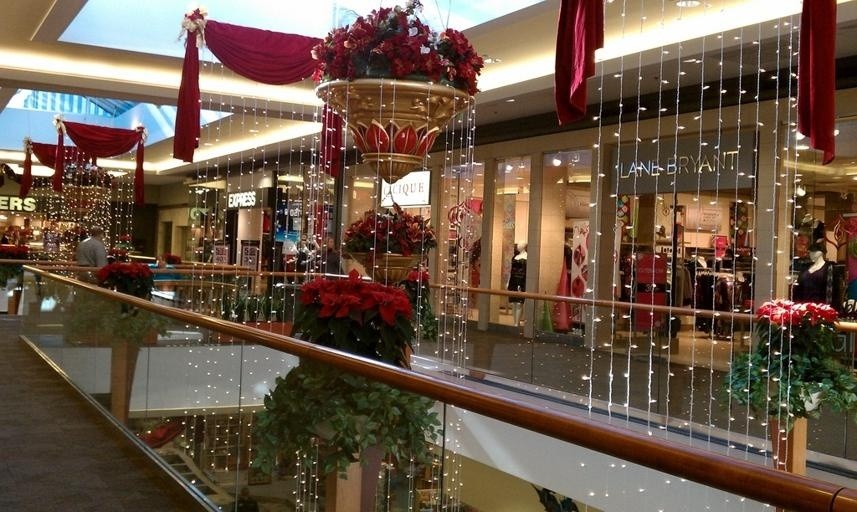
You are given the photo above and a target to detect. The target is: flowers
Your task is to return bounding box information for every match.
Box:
[299,264,430,334]
[101,258,156,298]
[344,207,436,253]
[720,299,857,417]
[312,6,482,101]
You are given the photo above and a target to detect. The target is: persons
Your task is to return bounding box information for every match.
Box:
[807,217,827,251]
[319,237,338,274]
[792,247,830,303]
[798,214,813,246]
[283,240,314,270]
[295,233,322,250]
[62,225,108,326]
[505,240,530,326]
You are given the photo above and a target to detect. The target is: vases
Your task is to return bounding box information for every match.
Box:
[349,251,427,288]
[772,415,807,477]
[312,81,476,183]
